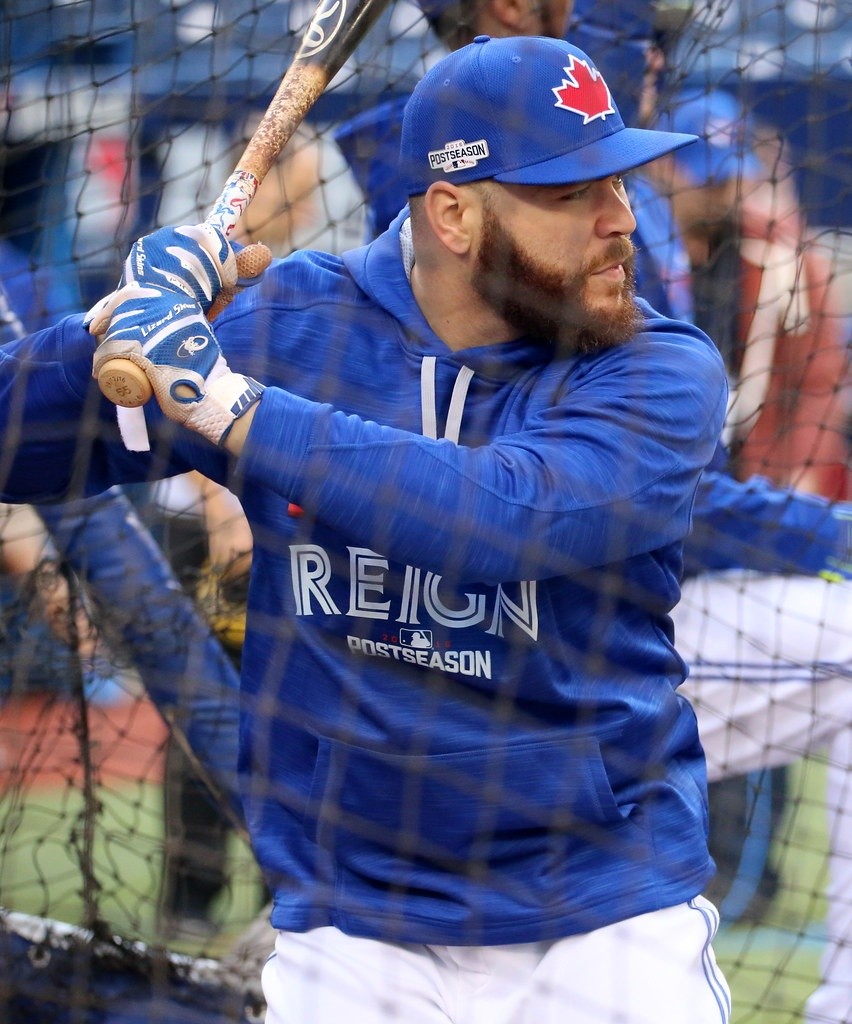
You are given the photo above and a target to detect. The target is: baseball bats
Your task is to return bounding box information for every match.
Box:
[98,0,390,408]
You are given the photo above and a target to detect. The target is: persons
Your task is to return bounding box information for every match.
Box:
[0,35,729,1023]
[0,1,851,1022]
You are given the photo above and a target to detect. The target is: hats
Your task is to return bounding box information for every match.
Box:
[653,87,763,187]
[399,33,698,199]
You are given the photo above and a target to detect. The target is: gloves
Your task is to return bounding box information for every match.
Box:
[121,222,274,306]
[85,291,264,448]
